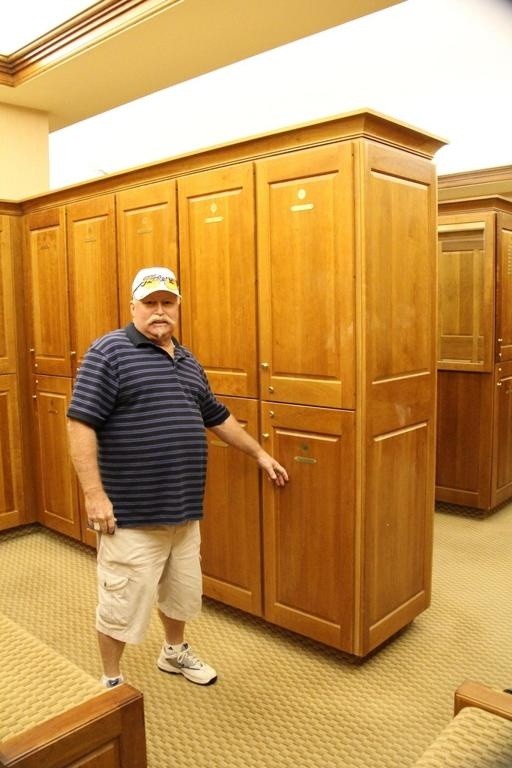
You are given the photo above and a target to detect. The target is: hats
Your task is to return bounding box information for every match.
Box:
[132,267,180,300]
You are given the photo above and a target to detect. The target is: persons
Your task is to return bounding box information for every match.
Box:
[67,267,287,691]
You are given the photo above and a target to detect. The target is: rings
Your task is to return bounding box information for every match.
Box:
[93,523,100,530]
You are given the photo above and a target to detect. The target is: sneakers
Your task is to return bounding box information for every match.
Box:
[156,641,217,685]
[106,678,122,687]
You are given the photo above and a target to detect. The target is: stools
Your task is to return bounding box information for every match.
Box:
[410,675,512,767]
[1,609,153,767]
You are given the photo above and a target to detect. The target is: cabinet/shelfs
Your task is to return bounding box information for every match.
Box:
[16,106,447,663]
[0,194,35,541]
[428,194,512,523]
